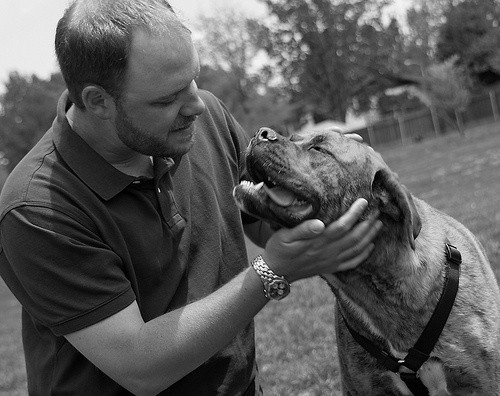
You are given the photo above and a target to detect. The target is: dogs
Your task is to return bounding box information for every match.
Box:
[234,128,500,396]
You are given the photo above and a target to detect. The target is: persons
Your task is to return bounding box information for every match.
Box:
[0,1,385,396]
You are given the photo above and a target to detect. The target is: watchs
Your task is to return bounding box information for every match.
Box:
[250,253,291,302]
